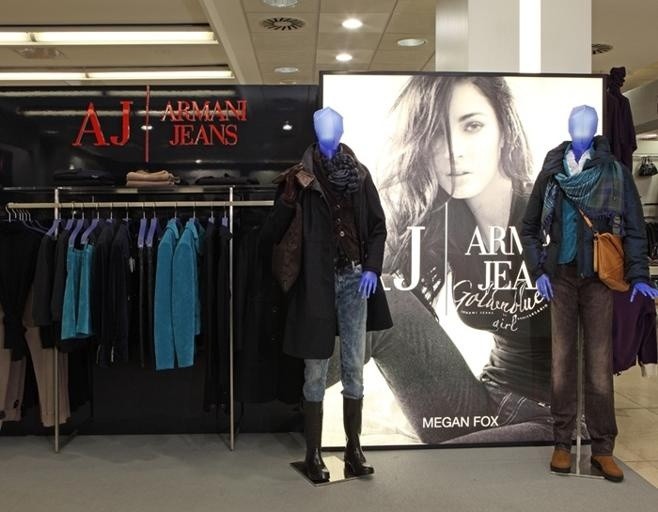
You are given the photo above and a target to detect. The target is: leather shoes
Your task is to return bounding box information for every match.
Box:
[550,445,572,473]
[590,455,624,483]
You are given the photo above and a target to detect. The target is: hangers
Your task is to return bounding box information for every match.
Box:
[59,199,229,248]
[4,201,32,222]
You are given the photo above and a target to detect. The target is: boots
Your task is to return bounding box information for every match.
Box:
[302,400,330,482]
[343,396,375,479]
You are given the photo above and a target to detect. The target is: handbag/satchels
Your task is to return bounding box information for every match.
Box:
[593,230,630,291]
[256,193,303,294]
[639,157,658,176]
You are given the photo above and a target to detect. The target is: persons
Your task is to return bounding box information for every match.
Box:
[520,103,658,483]
[268,106,392,483]
